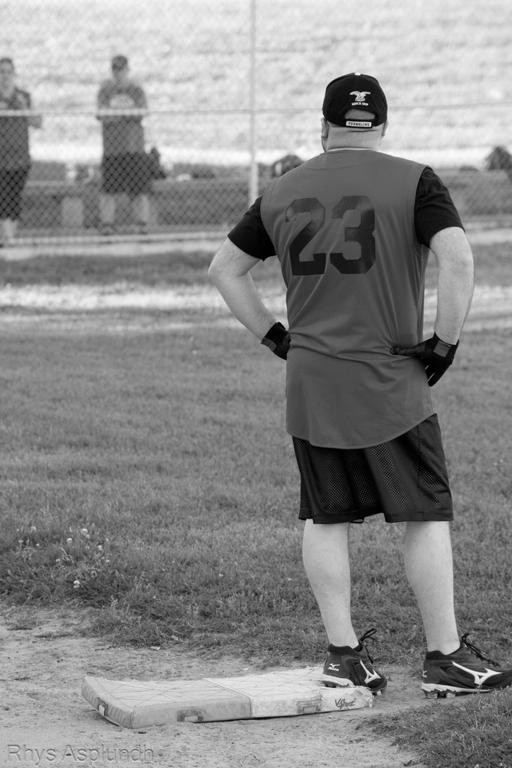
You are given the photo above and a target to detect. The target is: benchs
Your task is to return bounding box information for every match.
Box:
[20,168,512,227]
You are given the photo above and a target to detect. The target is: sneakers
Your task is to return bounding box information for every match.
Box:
[321,627,386,696]
[420,631,511,699]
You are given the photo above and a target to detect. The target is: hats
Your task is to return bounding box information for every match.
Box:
[321,71,388,129]
[112,56,127,69]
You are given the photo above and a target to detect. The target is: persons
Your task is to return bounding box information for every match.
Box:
[95,54,151,238]
[209,71,512,697]
[0,57,45,248]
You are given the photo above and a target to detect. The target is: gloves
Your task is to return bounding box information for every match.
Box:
[387,333,460,386]
[260,322,289,359]
